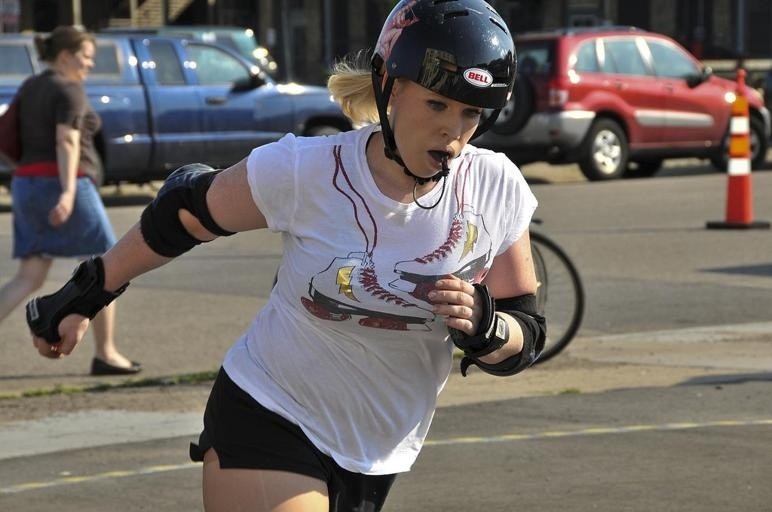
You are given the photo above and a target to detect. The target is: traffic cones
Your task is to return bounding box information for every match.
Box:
[704,68,772,230]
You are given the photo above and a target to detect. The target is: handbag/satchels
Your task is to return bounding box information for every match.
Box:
[1,97,21,164]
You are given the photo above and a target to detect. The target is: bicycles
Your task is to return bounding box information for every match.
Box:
[529,217,586,367]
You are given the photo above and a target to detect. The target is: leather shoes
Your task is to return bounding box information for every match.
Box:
[91,357,144,375]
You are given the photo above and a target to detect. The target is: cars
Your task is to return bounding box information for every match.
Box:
[1,28,360,197]
[469,23,771,180]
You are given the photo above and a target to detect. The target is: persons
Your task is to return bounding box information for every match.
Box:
[0,27,144,376]
[25,0,546,512]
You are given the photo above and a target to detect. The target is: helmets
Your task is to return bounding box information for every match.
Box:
[368,0,519,109]
